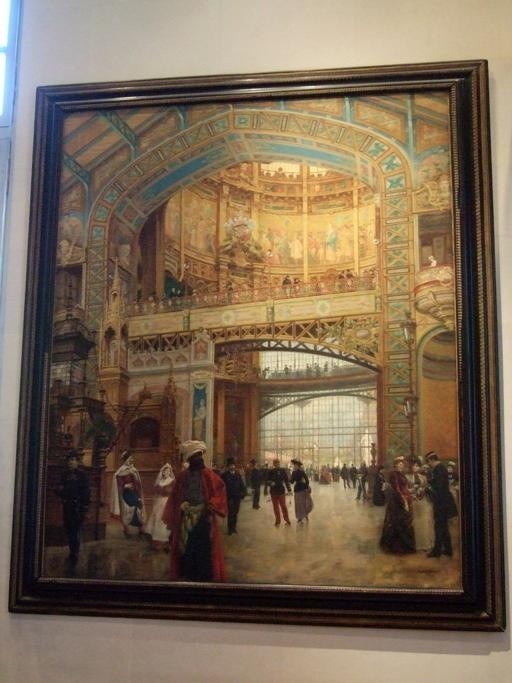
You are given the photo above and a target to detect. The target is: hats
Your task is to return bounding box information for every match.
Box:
[448,461,456,467]
[63,449,85,458]
[227,457,236,466]
[425,451,436,462]
[291,457,303,466]
[123,451,131,460]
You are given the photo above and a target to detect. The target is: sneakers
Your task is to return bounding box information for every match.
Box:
[228,528,237,535]
[426,549,452,557]
[124,529,147,536]
[276,520,291,527]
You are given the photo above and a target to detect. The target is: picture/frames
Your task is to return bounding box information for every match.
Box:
[8,57,502,634]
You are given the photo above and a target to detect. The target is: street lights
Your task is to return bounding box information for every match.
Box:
[398,307,419,472]
